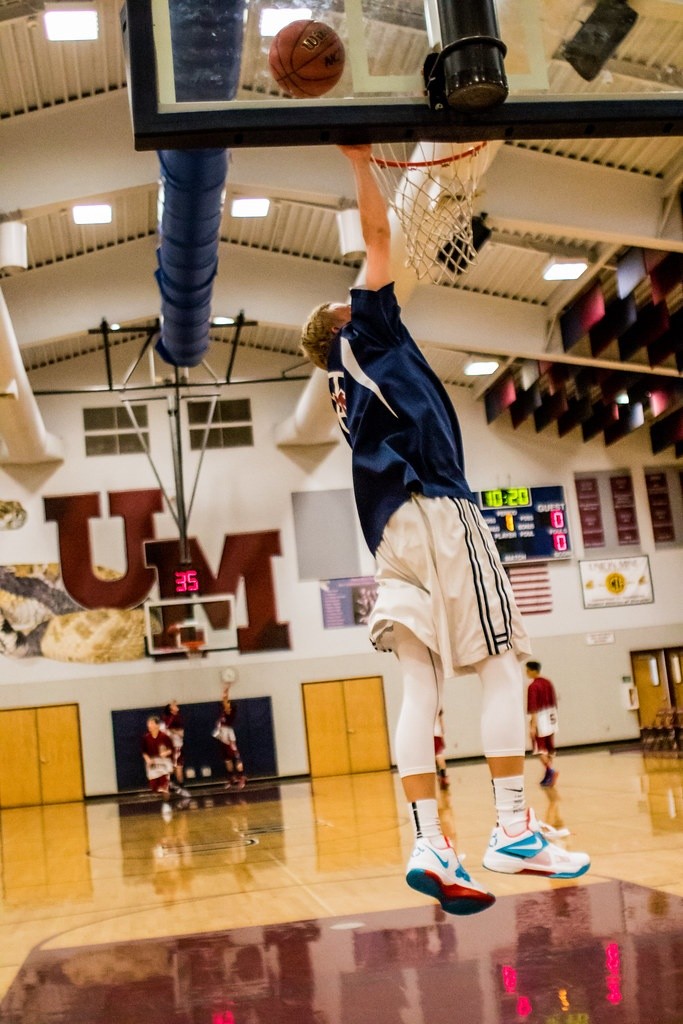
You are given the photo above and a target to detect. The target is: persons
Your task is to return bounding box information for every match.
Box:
[434,708,450,789]
[142,716,176,814]
[525,661,559,788]
[157,684,247,790]
[301,144,590,915]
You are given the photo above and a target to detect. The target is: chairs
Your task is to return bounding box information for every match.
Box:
[639,706,683,760]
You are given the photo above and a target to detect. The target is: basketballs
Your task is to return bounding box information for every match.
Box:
[268,19,344,99]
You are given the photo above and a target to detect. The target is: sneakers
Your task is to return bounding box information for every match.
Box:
[483,808,591,879]
[405,834,495,915]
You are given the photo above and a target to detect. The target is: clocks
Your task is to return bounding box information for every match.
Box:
[223,666,238,683]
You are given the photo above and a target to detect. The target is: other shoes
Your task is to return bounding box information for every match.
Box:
[541,769,557,786]
[162,803,171,812]
[440,777,447,790]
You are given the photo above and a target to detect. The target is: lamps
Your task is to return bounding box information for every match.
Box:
[563,0,640,82]
[229,194,272,218]
[420,0,511,111]
[40,2,101,41]
[541,254,589,282]
[462,354,501,377]
[72,202,115,225]
